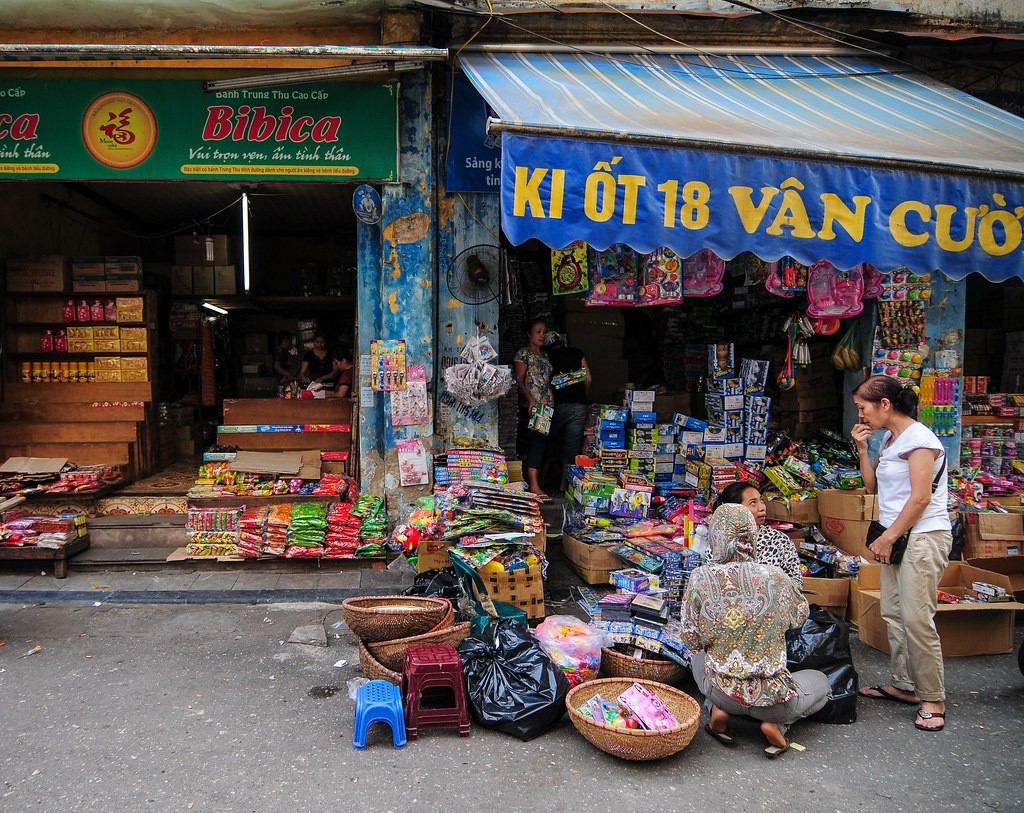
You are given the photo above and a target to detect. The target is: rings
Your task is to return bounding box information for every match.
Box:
[876,558,880,561]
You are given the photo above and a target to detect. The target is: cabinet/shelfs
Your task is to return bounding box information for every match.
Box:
[0,288,154,403]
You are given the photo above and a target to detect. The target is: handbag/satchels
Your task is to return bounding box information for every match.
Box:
[865,521,908,564]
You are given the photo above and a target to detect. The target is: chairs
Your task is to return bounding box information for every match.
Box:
[449,549,528,634]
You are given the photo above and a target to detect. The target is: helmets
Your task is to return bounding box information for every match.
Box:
[543,330,565,348]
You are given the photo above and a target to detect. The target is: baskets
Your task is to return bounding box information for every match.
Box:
[565,677,701,760]
[342,596,472,688]
[600,648,690,685]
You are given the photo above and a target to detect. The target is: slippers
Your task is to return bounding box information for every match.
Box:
[705,724,733,743]
[858,685,919,706]
[915,709,945,731]
[537,494,553,501]
[764,738,790,758]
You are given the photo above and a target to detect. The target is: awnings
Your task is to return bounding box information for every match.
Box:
[444,53,1024,283]
[1,43,449,91]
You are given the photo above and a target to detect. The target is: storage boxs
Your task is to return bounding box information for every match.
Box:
[415,525,545,618]
[171,234,236,295]
[0,253,148,383]
[559,347,1024,657]
[963,285,1024,392]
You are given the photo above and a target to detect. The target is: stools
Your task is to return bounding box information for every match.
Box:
[353,640,472,750]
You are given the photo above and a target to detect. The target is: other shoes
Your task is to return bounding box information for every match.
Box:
[557,488,567,497]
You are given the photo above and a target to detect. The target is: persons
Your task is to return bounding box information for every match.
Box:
[680,503,832,759]
[851,375,953,731]
[514,318,592,501]
[712,482,804,593]
[274,330,353,398]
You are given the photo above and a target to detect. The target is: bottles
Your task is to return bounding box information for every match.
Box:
[21,361,96,383]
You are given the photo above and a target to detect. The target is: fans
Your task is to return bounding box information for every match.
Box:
[447,244,511,306]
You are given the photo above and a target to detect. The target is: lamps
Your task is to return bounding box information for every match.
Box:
[201,301,228,314]
[241,189,250,292]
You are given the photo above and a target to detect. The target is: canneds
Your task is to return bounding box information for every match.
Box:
[21,362,95,383]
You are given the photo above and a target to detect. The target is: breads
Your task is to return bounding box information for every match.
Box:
[832,345,861,370]
